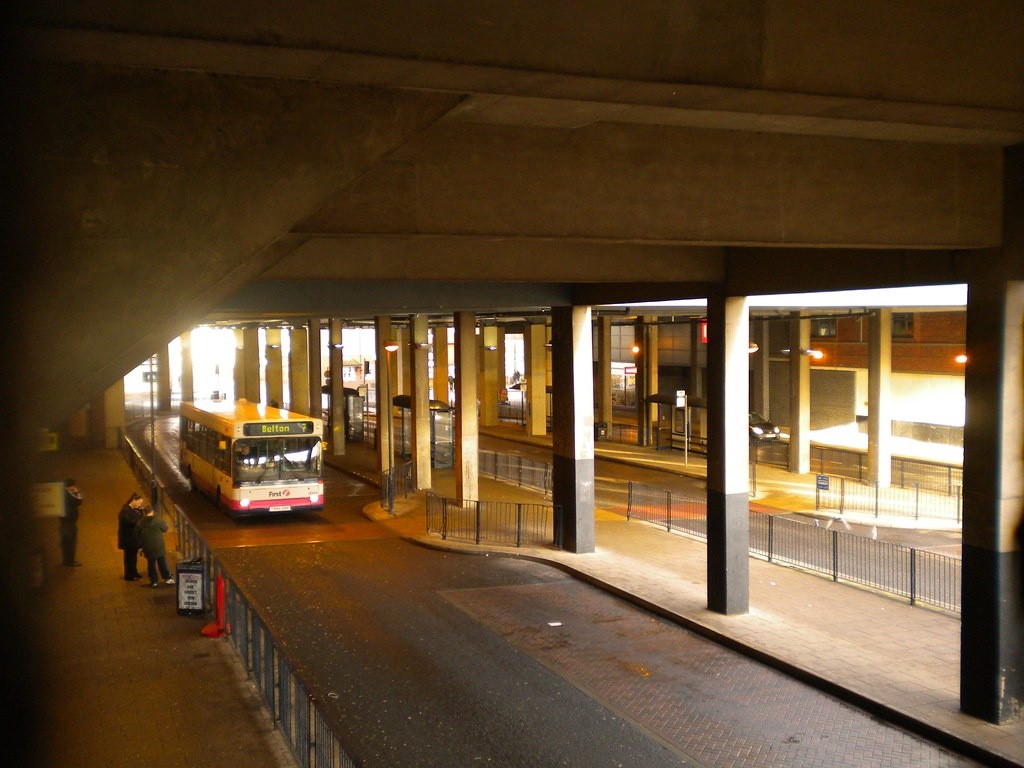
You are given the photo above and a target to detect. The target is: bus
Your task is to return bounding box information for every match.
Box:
[180,397,324,520]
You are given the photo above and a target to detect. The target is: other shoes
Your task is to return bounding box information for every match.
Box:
[73,562,81,567]
[132,575,143,581]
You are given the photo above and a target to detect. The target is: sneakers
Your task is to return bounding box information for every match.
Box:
[165,579,176,584]
[152,582,158,587]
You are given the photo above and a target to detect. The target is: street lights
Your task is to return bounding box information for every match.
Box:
[749,343,759,354]
[385,340,400,514]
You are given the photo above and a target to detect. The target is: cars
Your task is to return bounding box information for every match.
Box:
[749,412,780,442]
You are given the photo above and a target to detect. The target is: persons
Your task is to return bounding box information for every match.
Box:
[134,505,176,586]
[238,445,255,461]
[58,479,82,566]
[512,370,520,384]
[118,493,144,581]
[448,374,453,391]
